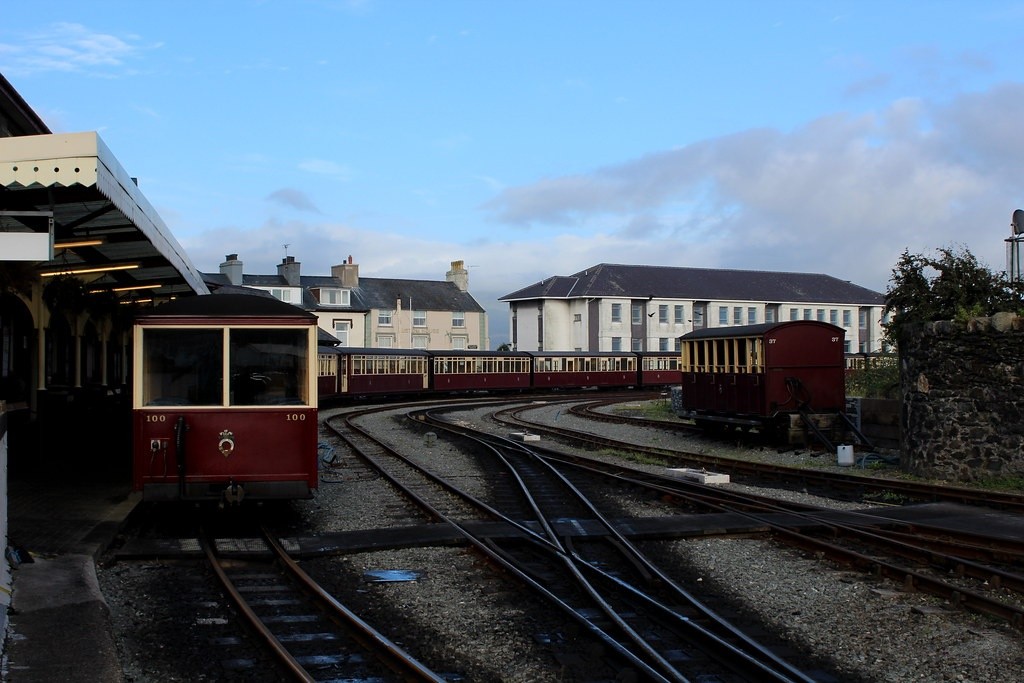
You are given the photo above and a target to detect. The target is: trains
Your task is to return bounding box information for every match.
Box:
[677,320,844,441]
[130,294,899,518]
